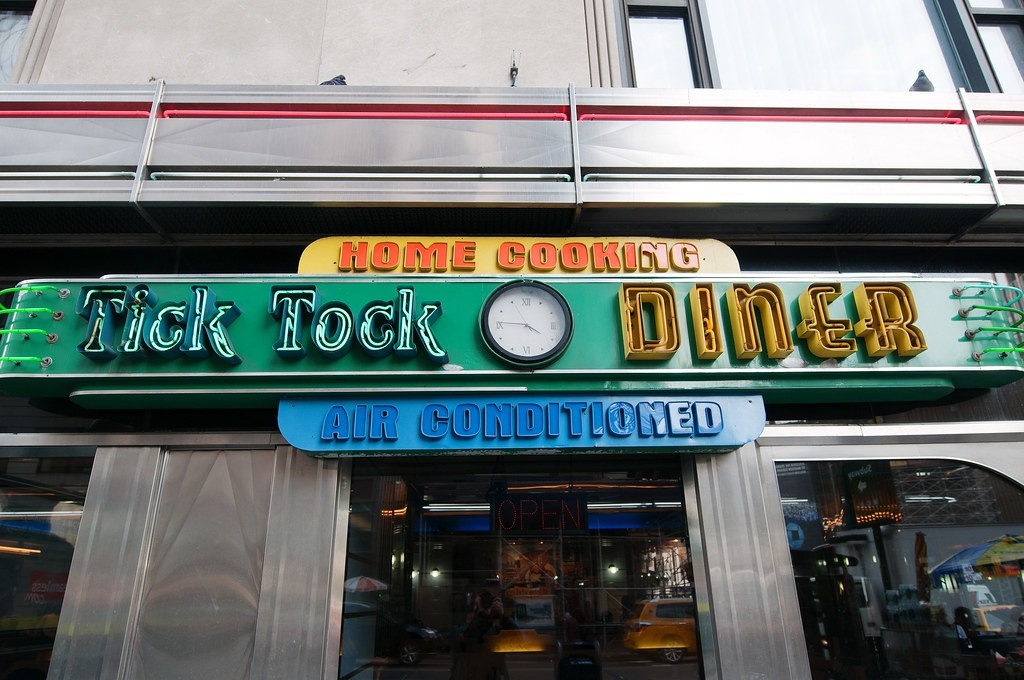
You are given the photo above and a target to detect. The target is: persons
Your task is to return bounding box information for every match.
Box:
[452,580,520,680]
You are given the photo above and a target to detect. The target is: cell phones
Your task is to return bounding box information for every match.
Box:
[493,597,501,602]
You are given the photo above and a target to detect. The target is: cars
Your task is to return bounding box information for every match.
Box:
[340,599,443,665]
[624,597,698,665]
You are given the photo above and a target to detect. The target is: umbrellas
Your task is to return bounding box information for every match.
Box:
[929,534,1024,586]
[343,576,387,593]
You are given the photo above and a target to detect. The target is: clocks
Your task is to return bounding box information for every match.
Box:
[482,282,571,362]
